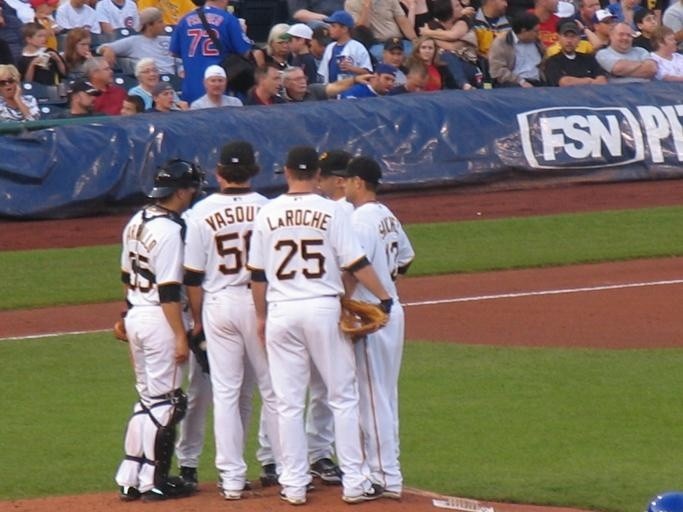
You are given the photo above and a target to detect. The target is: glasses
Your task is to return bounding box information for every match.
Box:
[0,80,15,87]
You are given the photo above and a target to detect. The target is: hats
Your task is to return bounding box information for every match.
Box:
[559,21,581,37]
[134,7,160,33]
[382,36,404,53]
[286,145,317,170]
[285,23,313,42]
[202,65,229,82]
[221,141,256,167]
[590,8,618,25]
[373,63,397,78]
[317,152,353,178]
[68,79,102,99]
[322,10,354,29]
[331,155,383,185]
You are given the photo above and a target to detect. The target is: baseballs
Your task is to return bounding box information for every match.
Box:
[476,211,482,215]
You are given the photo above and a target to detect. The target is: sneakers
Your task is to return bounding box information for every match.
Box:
[258,463,279,488]
[279,488,306,505]
[180,467,197,488]
[310,455,344,483]
[139,479,196,501]
[118,482,141,502]
[339,482,401,503]
[216,474,251,499]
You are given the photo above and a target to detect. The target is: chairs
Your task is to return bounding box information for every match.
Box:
[0,0,184,121]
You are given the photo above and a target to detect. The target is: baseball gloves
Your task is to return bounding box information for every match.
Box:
[340,298,391,339]
[186,327,209,373]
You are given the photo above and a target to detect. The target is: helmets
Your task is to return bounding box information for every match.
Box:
[145,161,203,199]
[647,491,683,512]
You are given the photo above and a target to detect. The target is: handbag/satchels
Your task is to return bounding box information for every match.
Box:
[220,53,255,90]
